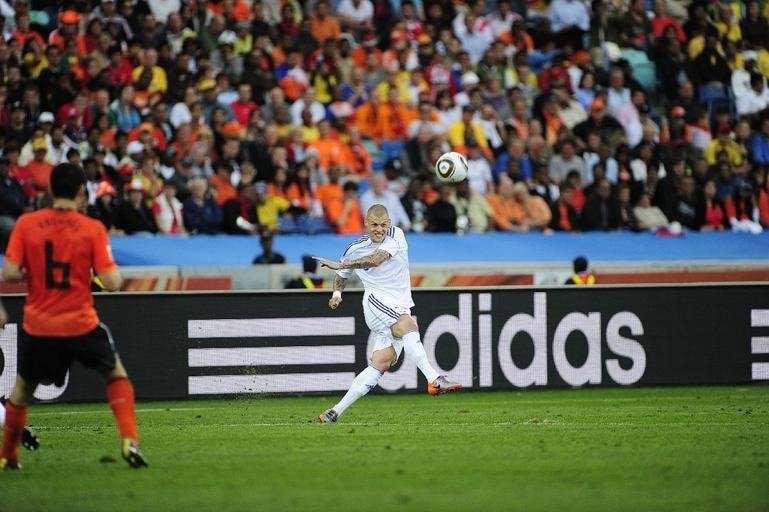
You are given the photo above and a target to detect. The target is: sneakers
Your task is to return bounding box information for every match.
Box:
[427,375,463,397]
[319,408,339,424]
[20,424,40,452]
[120,439,148,468]
[0,457,23,472]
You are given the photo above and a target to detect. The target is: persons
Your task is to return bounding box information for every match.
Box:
[564,255,599,285]
[280,251,332,289]
[306,201,464,426]
[1,305,41,451]
[1,161,151,472]
[249,235,289,266]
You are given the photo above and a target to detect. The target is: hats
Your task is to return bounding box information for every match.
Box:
[0,0,769,236]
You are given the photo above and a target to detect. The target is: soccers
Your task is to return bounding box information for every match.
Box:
[435,152,468,186]
